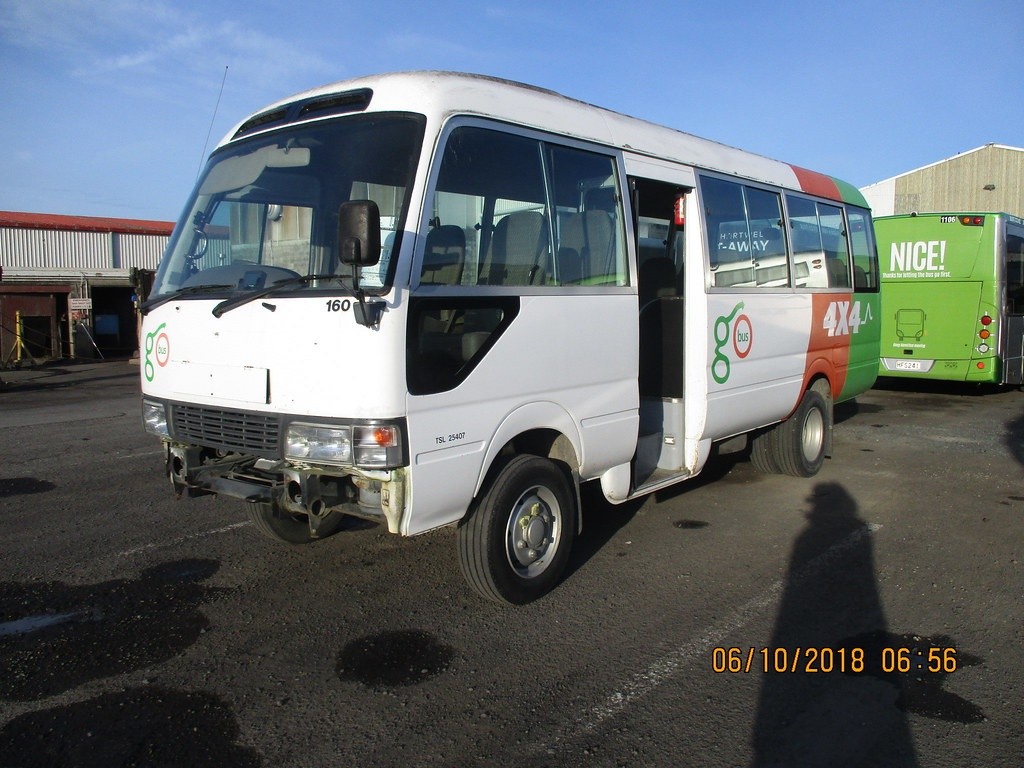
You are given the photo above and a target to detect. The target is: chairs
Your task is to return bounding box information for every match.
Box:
[827,258,847,288]
[559,210,617,287]
[855,265,867,287]
[416,225,467,332]
[476,210,549,285]
[639,256,676,299]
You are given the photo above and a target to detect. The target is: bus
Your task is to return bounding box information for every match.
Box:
[139,72,883,605]
[718,211,1024,384]
